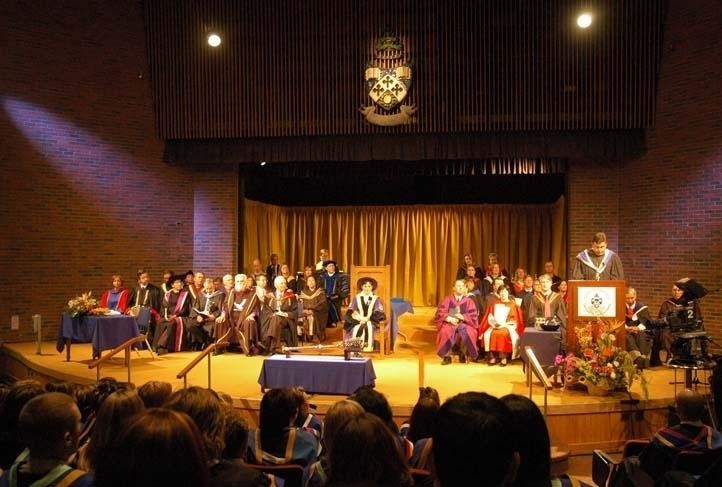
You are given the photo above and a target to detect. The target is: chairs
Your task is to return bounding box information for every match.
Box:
[592,427,649,486]
[670,445,708,479]
[408,461,434,487]
[243,461,306,482]
[340,264,392,361]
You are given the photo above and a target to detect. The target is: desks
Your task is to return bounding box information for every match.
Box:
[256,352,377,397]
[520,322,649,386]
[57,310,135,369]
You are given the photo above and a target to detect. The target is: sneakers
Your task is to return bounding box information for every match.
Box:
[501,358,507,364]
[489,358,497,364]
[157,347,168,354]
[312,338,320,344]
[258,341,268,351]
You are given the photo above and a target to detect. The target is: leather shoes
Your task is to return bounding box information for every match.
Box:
[459,351,465,362]
[331,322,336,327]
[441,356,451,365]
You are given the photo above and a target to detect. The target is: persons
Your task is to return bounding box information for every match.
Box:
[435,252,568,367]
[1,377,582,487]
[572,232,624,280]
[639,390,722,486]
[625,286,653,369]
[658,284,705,365]
[99,249,348,356]
[343,281,386,352]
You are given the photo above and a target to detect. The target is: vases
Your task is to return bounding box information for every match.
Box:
[584,381,611,397]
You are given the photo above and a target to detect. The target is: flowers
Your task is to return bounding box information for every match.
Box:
[546,318,649,397]
[67,294,97,314]
[336,336,367,353]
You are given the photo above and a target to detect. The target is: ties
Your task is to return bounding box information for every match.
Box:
[627,305,634,320]
[545,295,551,319]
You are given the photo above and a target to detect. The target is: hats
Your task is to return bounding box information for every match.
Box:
[181,271,193,278]
[323,261,336,266]
[357,277,377,291]
[167,275,185,288]
